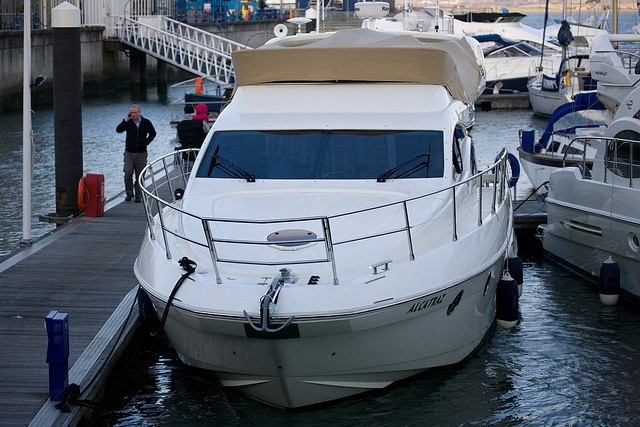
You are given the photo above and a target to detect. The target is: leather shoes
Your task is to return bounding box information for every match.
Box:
[125,194,134,201]
[136,197,141,202]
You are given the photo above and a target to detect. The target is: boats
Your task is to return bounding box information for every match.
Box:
[360,5,627,98]
[133,30,522,413]
[527,31,631,117]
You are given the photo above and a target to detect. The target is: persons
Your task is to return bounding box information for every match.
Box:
[188,104,210,122]
[116,104,157,203]
[176,106,210,191]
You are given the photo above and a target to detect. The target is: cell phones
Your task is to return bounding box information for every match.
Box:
[128,113,131,117]
